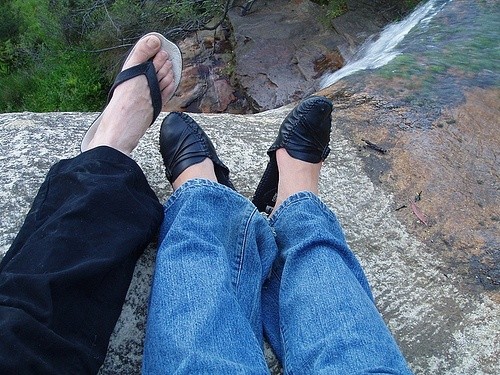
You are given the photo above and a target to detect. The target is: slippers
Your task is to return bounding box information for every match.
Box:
[158,112,238,193]
[79,30,184,155]
[253,96,333,214]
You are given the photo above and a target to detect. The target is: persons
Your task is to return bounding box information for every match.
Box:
[0,32,182,375]
[141,94,420,374]
